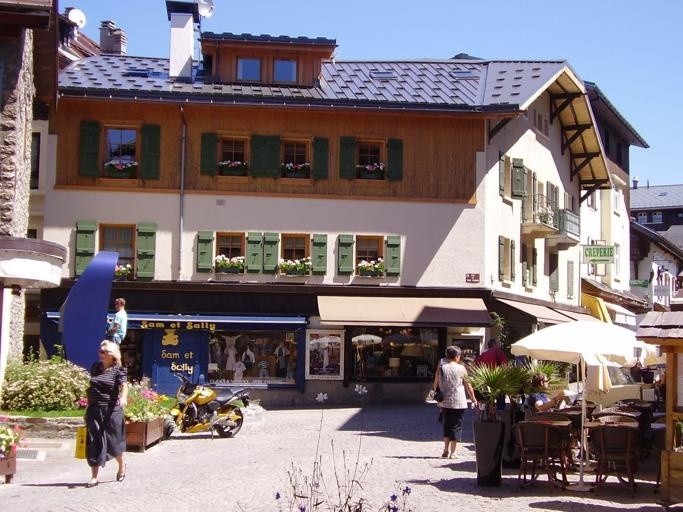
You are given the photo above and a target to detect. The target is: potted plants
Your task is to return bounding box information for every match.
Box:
[461,359,567,487]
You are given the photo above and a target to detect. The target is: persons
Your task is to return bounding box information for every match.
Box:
[477,338,508,370]
[432,348,448,424]
[83,339,128,488]
[106,297,128,348]
[241,348,256,376]
[355,339,365,378]
[273,341,289,378]
[223,343,238,381]
[257,355,272,377]
[429,345,477,461]
[526,372,588,469]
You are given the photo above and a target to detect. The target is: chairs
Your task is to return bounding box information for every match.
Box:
[513,399,655,498]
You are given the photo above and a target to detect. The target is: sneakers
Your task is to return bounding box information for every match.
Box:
[442,449,460,459]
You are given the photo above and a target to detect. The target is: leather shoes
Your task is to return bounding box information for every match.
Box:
[115,462,127,482]
[86,478,99,488]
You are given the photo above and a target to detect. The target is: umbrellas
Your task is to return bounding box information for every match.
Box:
[508,317,658,486]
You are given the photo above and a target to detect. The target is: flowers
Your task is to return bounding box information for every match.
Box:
[355,162,385,176]
[280,161,311,176]
[215,254,245,271]
[277,256,312,273]
[123,376,169,423]
[0,416,25,459]
[218,160,249,173]
[104,159,139,174]
[356,257,385,273]
[114,263,132,279]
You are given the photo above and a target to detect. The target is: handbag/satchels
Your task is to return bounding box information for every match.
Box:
[424,387,444,404]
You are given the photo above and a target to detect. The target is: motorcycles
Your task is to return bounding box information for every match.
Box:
[163,373,250,440]
